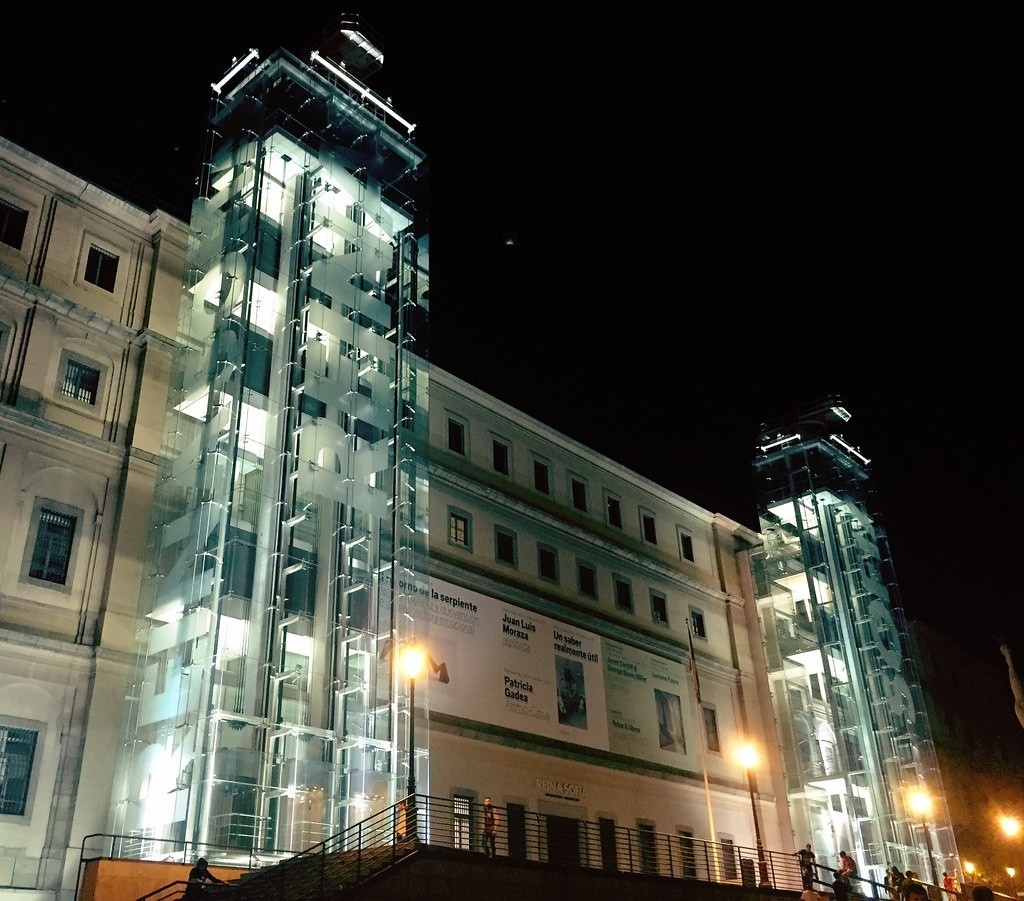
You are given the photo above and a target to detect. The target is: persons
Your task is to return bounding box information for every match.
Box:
[396,801,407,843]
[481,797,500,859]
[181,857,229,900]
[793,843,819,890]
[801,850,995,901]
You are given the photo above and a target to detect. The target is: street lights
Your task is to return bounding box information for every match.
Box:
[736,743,775,888]
[910,792,942,900]
[401,649,426,843]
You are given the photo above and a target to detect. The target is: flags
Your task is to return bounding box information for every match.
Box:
[686,625,703,706]
